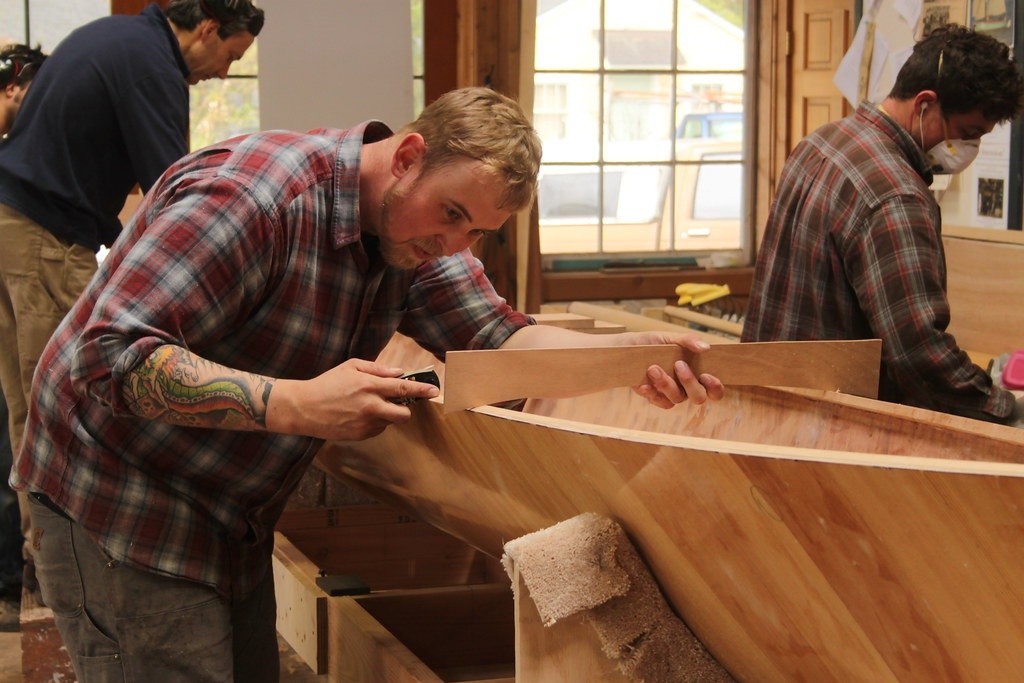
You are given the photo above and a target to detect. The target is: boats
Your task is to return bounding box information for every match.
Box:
[313,315,1024,683]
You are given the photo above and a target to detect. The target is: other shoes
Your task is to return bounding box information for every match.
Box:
[0,601,20,631]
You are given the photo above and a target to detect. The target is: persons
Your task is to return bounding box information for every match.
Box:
[7,85,729,682]
[1,1,267,594]
[739,23,1024,432]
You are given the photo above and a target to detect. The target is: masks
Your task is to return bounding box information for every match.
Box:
[916,107,981,174]
